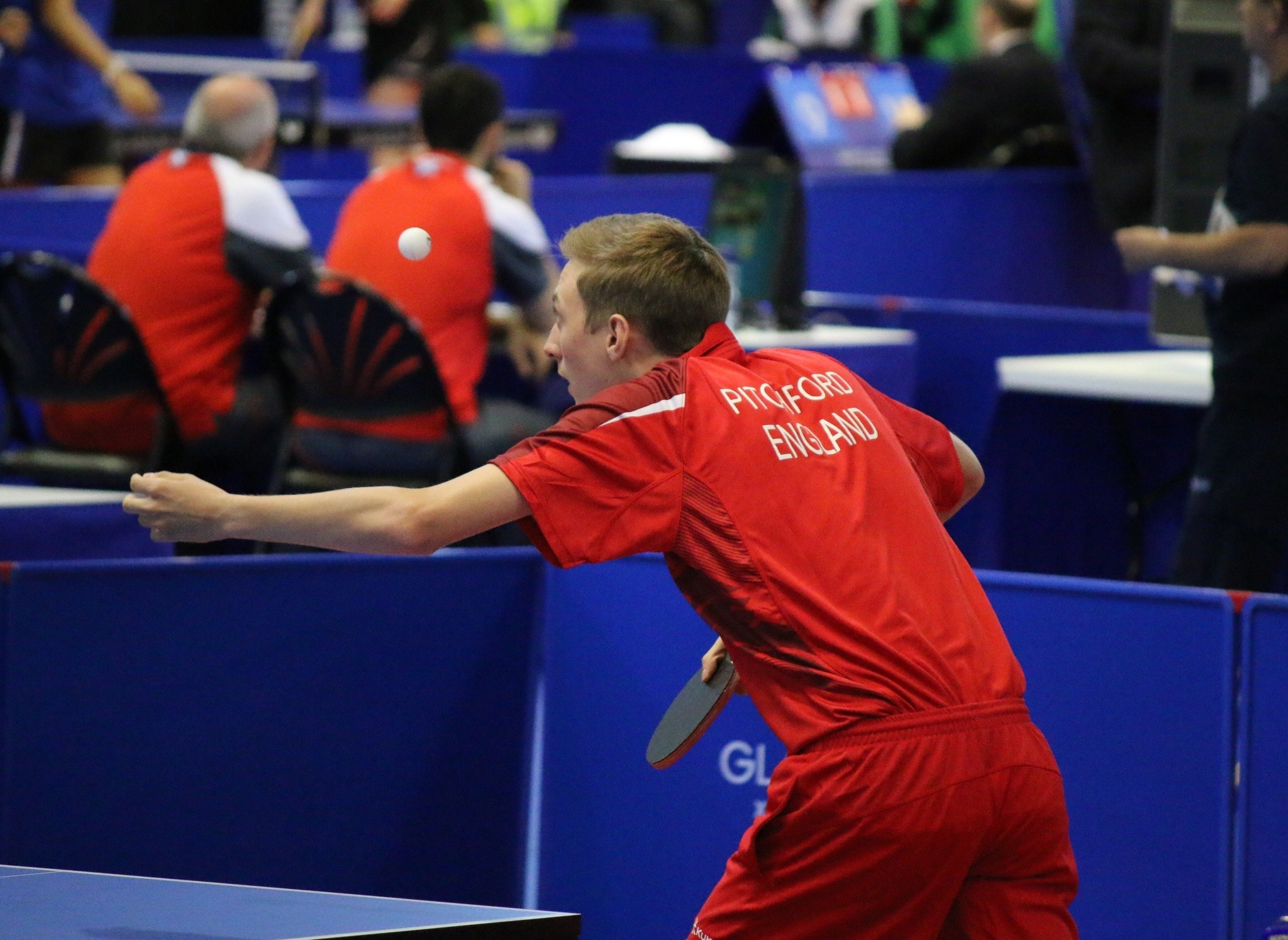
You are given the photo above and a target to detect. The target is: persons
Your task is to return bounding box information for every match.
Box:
[125,209,1081,940]
[0,1,1288,594]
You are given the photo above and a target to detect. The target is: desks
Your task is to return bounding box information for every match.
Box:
[81,85,567,168]
[725,320,915,354]
[988,340,1223,588]
[264,266,479,499]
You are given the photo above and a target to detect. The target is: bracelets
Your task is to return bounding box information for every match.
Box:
[103,56,125,87]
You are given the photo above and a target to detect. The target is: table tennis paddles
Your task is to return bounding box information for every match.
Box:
[643,651,742,769]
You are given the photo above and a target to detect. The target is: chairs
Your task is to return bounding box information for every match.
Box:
[0,250,263,561]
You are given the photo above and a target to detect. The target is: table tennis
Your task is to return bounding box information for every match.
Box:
[396,227,433,263]
